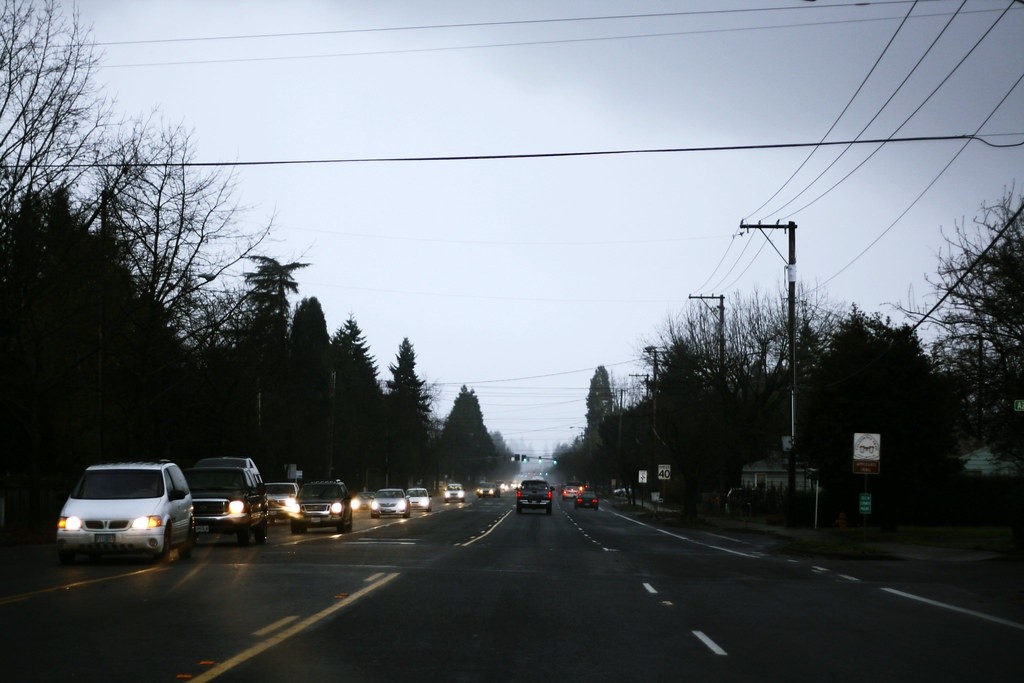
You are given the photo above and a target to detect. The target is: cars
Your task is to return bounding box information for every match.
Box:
[263,481,300,523]
[355,491,377,509]
[561,479,586,500]
[405,488,431,512]
[291,478,355,534]
[444,483,465,504]
[369,486,410,521]
[476,480,501,498]
[573,491,600,509]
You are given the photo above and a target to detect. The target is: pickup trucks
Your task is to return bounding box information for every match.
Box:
[514,477,555,515]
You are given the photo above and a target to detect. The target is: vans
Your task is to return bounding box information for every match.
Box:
[56,459,195,564]
[181,456,270,547]
